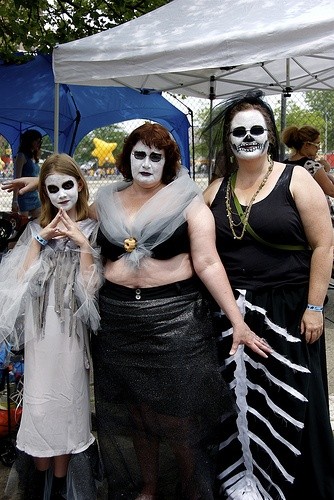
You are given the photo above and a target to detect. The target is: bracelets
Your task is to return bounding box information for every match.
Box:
[307,304,323,311]
[35,234,48,246]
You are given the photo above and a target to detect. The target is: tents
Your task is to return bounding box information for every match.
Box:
[52,0,334,163]
[0,52,195,181]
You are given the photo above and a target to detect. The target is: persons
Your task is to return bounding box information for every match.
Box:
[1,122,274,500]
[11,129,41,217]
[0,152,105,500]
[196,89,334,500]
[282,126,334,198]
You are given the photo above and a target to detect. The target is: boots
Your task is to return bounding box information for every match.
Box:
[50,470,67,500]
[24,472,48,500]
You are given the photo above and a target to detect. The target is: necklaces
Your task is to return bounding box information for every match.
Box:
[225,160,274,239]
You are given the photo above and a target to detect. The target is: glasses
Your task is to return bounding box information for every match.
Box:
[304,141,320,148]
